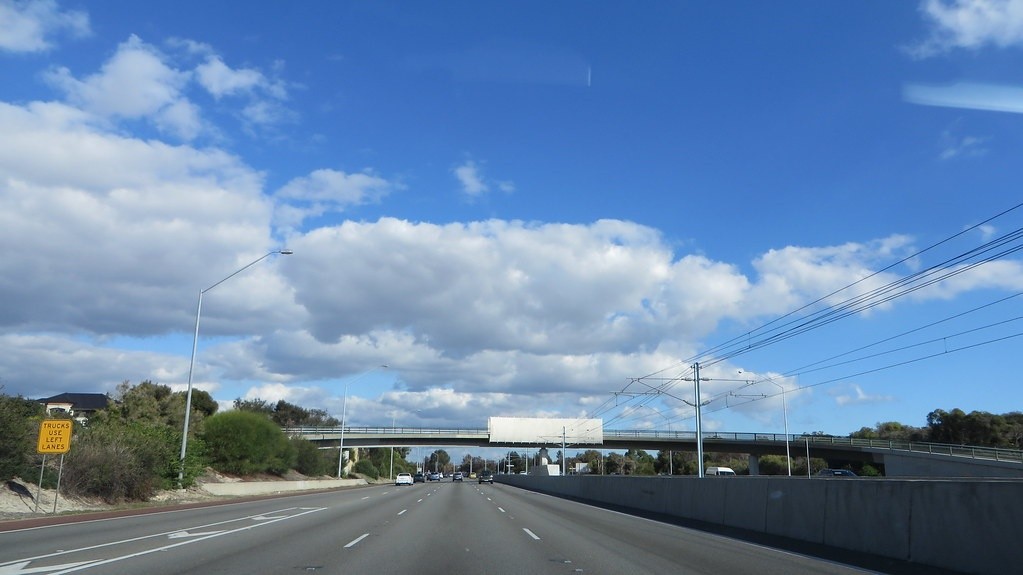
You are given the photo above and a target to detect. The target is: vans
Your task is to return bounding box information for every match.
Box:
[705,467,736,476]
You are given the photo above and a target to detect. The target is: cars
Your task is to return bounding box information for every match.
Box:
[427,474,431,480]
[439,473,443,478]
[478,471,494,484]
[818,469,856,477]
[430,472,440,481]
[414,474,425,483]
[395,473,414,486]
[470,473,476,479]
[520,471,527,475]
[453,472,463,482]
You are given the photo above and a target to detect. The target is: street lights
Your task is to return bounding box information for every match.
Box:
[177,249,293,488]
[338,365,388,480]
[484,459,486,471]
[432,452,438,462]
[390,410,421,480]
[739,371,791,476]
